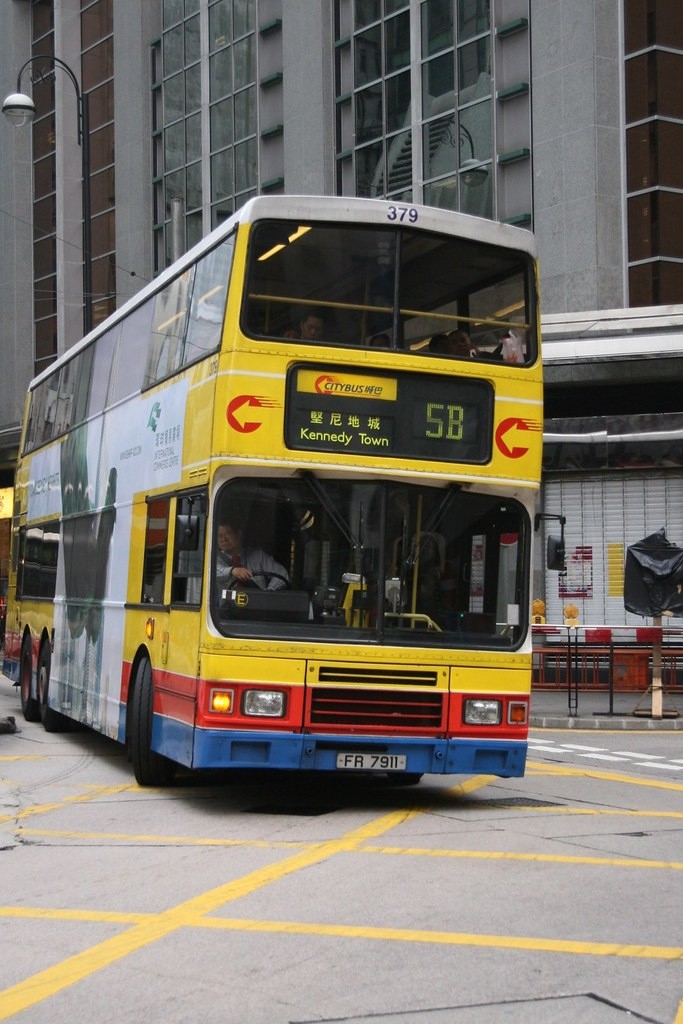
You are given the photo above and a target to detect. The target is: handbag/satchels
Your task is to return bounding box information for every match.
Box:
[503,329,525,363]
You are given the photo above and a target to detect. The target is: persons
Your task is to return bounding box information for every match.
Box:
[448,329,471,357]
[282,326,301,340]
[429,334,450,355]
[300,309,326,340]
[215,519,288,594]
[370,334,390,348]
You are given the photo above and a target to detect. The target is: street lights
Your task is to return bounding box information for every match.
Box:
[2,55,94,337]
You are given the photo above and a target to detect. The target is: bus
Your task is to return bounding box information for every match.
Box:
[2,194,566,793]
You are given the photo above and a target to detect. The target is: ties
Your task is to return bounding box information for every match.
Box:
[232,556,240,588]
